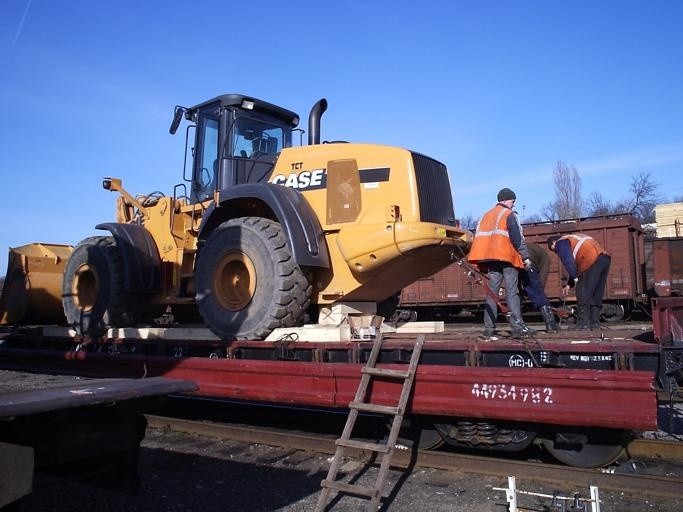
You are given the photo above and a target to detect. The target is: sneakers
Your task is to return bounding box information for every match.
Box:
[567,324,600,331]
[511,326,538,336]
[483,329,495,336]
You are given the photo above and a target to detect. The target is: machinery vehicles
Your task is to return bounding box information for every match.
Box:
[0,87,475,341]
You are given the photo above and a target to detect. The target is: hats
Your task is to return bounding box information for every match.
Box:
[495,187,517,202]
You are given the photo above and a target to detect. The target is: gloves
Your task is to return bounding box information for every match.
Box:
[523,258,532,271]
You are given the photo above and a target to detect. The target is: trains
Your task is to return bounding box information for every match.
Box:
[638,234,682,303]
[393,210,646,325]
[0,322,666,475]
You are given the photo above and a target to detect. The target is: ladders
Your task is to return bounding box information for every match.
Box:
[314,333,424,512]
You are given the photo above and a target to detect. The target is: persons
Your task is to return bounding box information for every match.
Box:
[518,242,562,333]
[545,233,613,332]
[465,188,537,339]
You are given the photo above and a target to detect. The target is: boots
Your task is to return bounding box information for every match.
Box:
[540,305,562,333]
